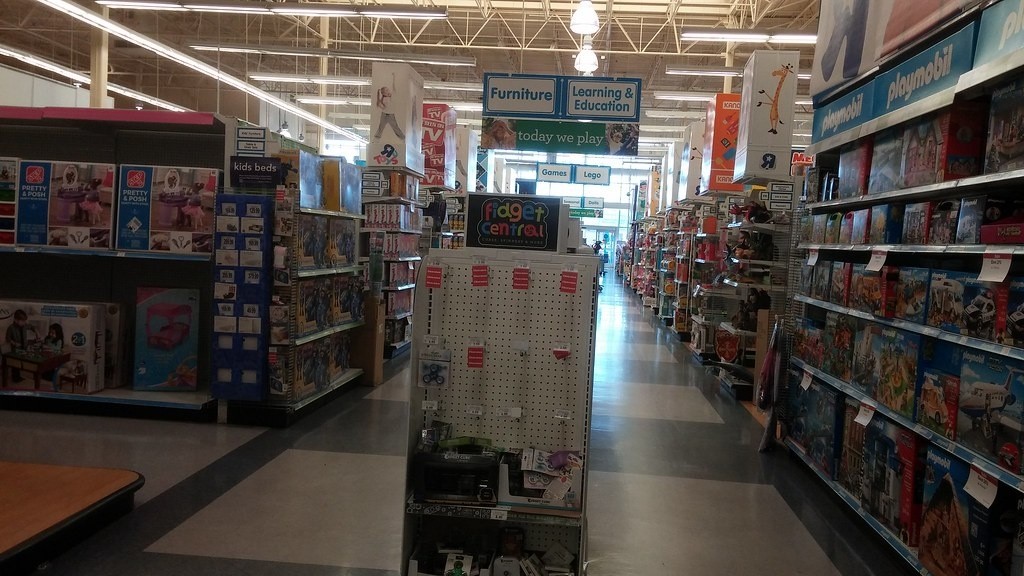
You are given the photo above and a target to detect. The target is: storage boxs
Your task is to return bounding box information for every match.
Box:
[0,300,107,393]
[401,495,583,576]
[64,298,128,391]
[0,157,21,247]
[778,74,1024,576]
[658,294,669,316]
[292,159,466,415]
[114,164,224,256]
[658,271,673,294]
[14,158,119,250]
[133,286,200,393]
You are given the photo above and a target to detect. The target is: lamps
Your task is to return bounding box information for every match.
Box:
[299,119,304,142]
[0,0,817,151]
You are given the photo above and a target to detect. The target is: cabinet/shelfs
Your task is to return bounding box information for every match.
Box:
[0,242,213,416]
[357,195,427,358]
[614,187,794,399]
[443,194,466,234]
[778,1,1023,576]
[417,184,457,235]
[292,189,364,414]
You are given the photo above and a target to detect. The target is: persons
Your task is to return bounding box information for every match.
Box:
[181,182,205,229]
[0,166,9,181]
[482,120,515,149]
[79,178,104,223]
[372,73,405,143]
[35,323,64,388]
[605,124,638,156]
[5,310,40,384]
